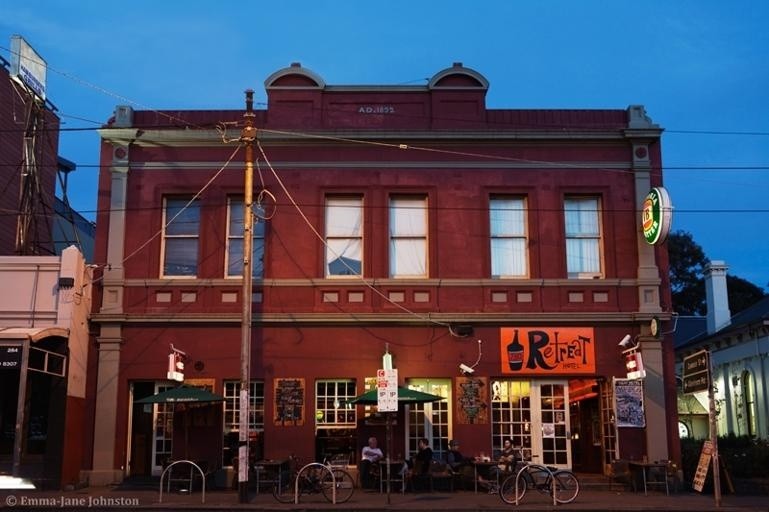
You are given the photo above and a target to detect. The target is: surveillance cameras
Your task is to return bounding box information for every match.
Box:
[618,334,631,348]
[459,363,475,374]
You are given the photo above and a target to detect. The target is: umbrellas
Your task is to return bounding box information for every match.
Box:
[137,381,225,458]
[348,384,445,504]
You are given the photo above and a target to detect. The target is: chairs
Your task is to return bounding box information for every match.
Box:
[410,456,466,493]
[609,458,637,494]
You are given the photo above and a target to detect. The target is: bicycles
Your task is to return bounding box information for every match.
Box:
[500,446,580,505]
[271,452,354,503]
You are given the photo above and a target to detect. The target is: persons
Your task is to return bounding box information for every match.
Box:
[449,438,498,495]
[483,439,516,479]
[401,437,432,493]
[360,436,385,490]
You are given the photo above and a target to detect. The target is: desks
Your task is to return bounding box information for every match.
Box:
[167,461,198,493]
[469,459,507,493]
[629,460,670,497]
[255,459,287,496]
[378,460,406,496]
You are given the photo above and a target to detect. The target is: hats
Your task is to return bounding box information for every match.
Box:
[447,439,461,445]
